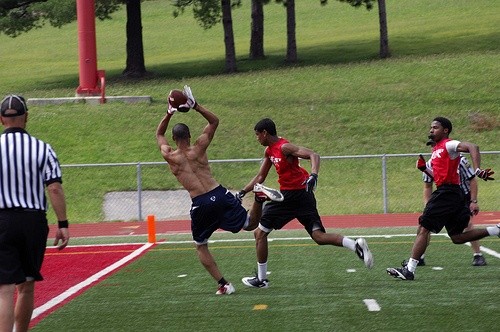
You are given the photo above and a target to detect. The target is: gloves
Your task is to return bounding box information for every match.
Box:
[236,189,246,203]
[416,154,427,171]
[179,85,198,110]
[301,173,318,194]
[166,102,177,117]
[474,169,495,182]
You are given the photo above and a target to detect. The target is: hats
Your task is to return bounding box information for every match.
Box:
[426,139,435,146]
[0,94,26,117]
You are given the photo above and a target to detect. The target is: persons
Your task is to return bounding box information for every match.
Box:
[156,85,284,296]
[0,95,69,332]
[236,118,374,288]
[386,116,500,280]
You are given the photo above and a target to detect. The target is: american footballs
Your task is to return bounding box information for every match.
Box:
[167,89,191,113]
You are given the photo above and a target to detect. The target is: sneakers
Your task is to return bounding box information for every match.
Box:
[386,260,415,280]
[252,184,285,202]
[472,256,487,266]
[401,258,425,267]
[355,238,374,268]
[215,283,236,296]
[241,270,269,290]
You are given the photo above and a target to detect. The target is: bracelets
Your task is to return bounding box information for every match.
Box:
[471,200,477,203]
[58,220,68,228]
